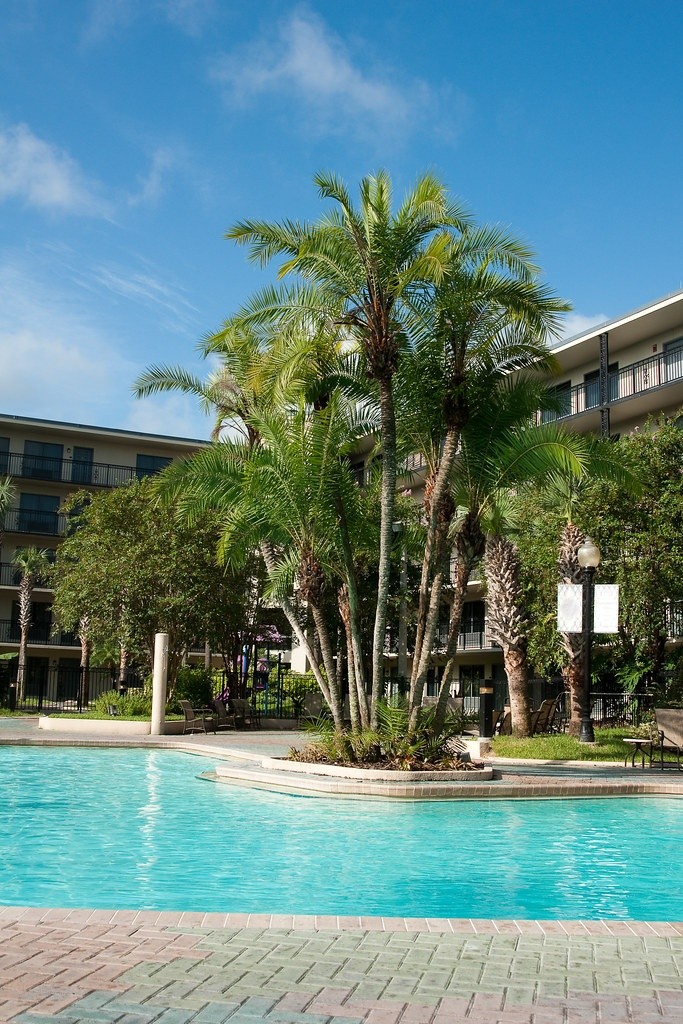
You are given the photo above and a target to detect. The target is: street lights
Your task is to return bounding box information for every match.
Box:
[576,532,600,744]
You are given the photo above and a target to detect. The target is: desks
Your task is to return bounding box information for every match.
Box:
[622,738,653,768]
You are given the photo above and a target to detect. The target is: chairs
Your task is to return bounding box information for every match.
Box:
[178,698,261,736]
[488,695,561,736]
[298,692,464,738]
